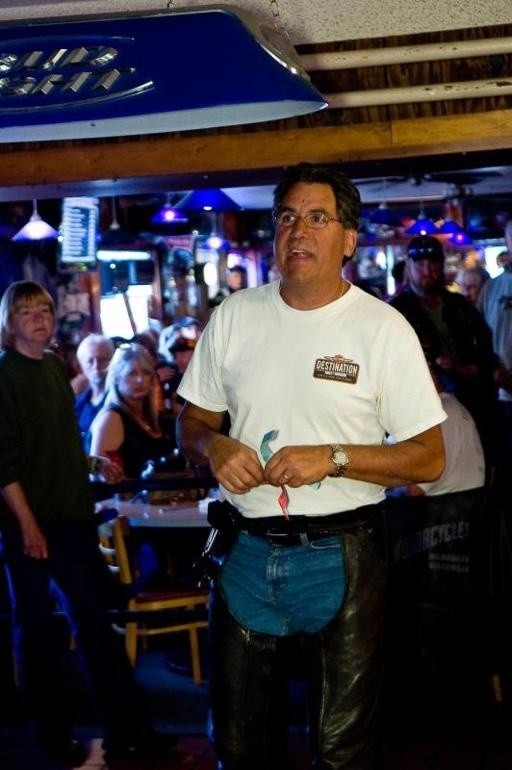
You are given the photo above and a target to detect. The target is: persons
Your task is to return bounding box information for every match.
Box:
[0,227,512,770]
[176,163,450,770]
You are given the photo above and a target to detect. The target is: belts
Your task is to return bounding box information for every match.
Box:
[240,519,368,546]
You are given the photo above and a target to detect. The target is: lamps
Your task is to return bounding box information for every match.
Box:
[57,222,101,245]
[108,195,120,230]
[151,194,188,222]
[171,189,244,211]
[369,178,406,227]
[405,178,442,235]
[12,200,61,247]
[438,178,466,236]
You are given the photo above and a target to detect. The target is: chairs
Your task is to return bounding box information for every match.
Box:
[98,516,209,685]
[375,464,505,710]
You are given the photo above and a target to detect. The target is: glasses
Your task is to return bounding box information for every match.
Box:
[274,211,353,228]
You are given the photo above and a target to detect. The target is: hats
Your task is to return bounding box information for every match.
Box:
[407,235,443,261]
